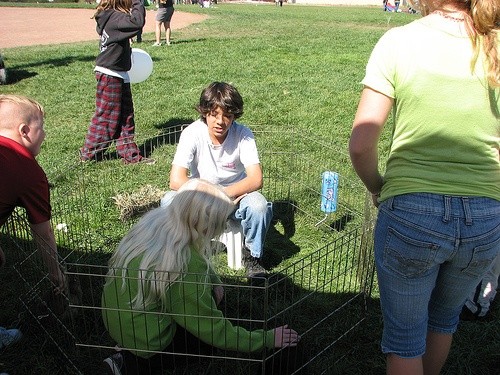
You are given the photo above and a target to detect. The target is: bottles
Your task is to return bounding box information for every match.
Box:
[321,171,338,213]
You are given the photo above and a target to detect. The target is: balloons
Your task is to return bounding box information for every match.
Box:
[126,49,153,84]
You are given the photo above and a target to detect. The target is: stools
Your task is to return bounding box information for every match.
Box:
[210,218,244,270]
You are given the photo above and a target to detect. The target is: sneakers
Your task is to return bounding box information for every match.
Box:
[103,354,122,375]
[0,373,9,375]
[0,327,23,349]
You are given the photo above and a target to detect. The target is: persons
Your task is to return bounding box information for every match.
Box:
[97,178,302,375]
[78,0,146,163]
[161,82,274,280]
[0,93,67,375]
[347,0,500,375]
[152,0,174,47]
[459,255,500,320]
[382,0,388,12]
[394,0,400,13]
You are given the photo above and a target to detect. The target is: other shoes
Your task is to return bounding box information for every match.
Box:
[124,157,156,166]
[98,155,108,162]
[460,309,485,322]
[152,42,161,47]
[166,42,171,46]
